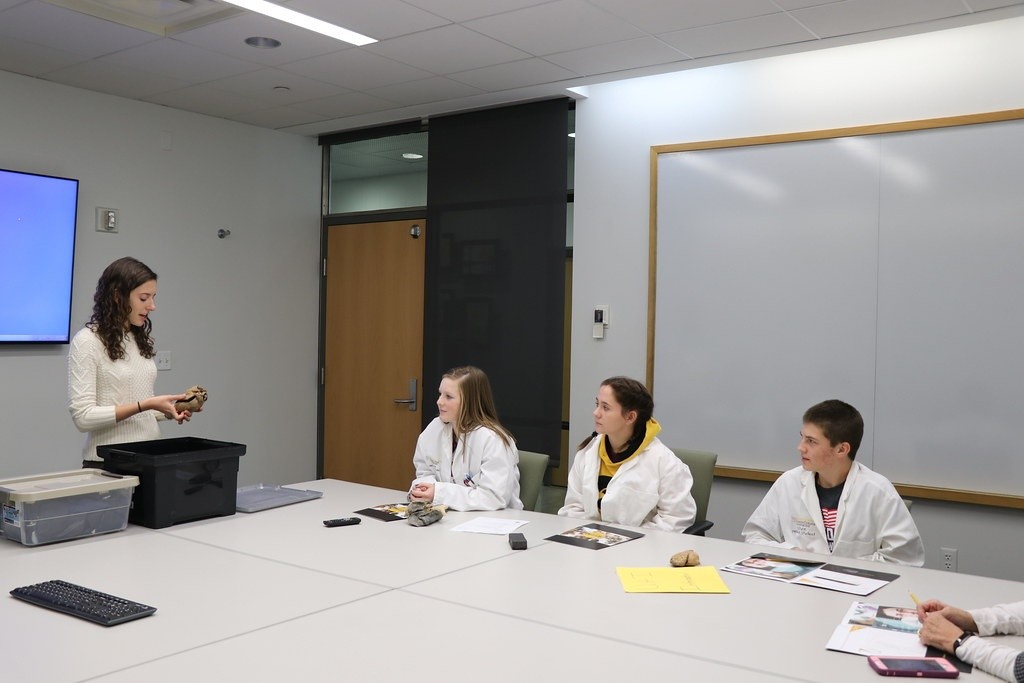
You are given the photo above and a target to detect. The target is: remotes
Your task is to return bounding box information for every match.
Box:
[323,517,361,527]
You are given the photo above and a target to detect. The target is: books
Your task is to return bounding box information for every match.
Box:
[721,552,901,597]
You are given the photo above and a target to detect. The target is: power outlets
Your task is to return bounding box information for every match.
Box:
[154,351,171,370]
[939,548,958,572]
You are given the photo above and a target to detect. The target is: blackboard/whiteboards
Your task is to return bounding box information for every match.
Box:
[644,108,1023,511]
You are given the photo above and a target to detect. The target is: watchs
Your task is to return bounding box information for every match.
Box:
[954,629,975,655]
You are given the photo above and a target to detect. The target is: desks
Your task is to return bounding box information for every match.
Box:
[0,478,1023,683]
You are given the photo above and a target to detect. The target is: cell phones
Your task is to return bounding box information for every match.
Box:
[868,656,960,679]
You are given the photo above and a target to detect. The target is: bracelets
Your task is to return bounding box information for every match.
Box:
[138,401,142,412]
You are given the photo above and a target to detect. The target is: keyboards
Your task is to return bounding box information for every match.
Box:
[9,580,157,626]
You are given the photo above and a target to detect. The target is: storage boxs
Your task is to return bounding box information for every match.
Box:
[96,437,247,530]
[0,469,140,546]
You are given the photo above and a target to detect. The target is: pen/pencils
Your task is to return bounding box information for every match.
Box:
[906,588,921,606]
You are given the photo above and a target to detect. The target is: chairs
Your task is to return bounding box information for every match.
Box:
[517,450,550,510]
[666,448,718,537]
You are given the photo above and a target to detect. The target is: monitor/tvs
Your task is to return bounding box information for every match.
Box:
[0,169,79,345]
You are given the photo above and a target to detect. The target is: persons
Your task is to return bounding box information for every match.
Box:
[558,378,697,534]
[68,257,205,468]
[877,606,917,621]
[742,399,926,567]
[916,598,1024,682]
[408,366,525,511]
